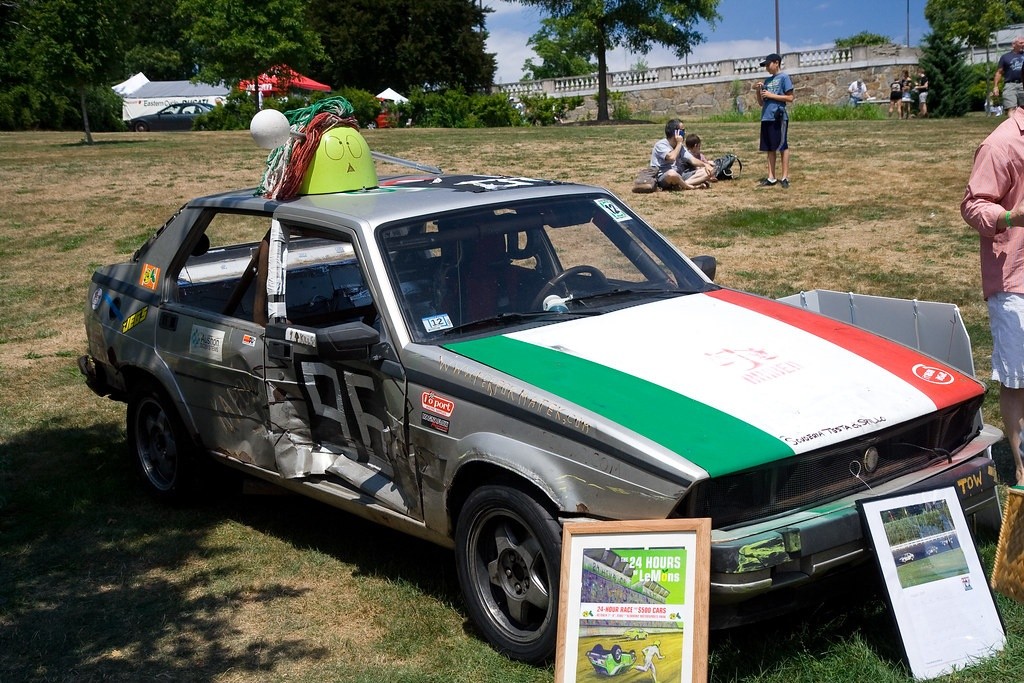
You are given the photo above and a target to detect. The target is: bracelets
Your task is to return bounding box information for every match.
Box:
[1006,211,1012,227]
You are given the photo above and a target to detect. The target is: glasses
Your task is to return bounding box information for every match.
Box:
[678,129,685,132]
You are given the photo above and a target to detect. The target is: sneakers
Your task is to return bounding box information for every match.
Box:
[761,177,777,186]
[779,178,790,192]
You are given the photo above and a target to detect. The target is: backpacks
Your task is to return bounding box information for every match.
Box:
[713,154,742,180]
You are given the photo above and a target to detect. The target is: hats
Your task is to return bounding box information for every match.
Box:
[857,78,863,83]
[759,54,782,67]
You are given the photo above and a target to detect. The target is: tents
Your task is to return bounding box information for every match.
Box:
[373,87,408,105]
[235,63,331,110]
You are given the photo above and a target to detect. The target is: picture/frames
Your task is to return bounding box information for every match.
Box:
[554,518,711,683]
[855,482,1007,683]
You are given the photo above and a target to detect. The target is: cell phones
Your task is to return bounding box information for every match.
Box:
[672,129,686,137]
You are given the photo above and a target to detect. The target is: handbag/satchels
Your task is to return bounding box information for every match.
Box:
[632,167,659,193]
[984,92,1003,118]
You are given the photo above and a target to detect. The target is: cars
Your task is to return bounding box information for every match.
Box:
[75,170,1008,668]
[899,552,914,563]
[940,537,953,546]
[363,97,400,130]
[925,545,939,556]
[131,102,217,133]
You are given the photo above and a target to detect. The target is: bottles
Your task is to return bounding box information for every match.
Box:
[762,84,768,101]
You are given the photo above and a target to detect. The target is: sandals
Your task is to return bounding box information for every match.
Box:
[709,177,718,182]
[694,182,709,189]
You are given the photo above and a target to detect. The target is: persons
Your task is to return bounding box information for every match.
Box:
[846,78,869,107]
[961,61,1024,490]
[752,53,795,188]
[650,118,718,192]
[888,67,928,120]
[993,36,1024,118]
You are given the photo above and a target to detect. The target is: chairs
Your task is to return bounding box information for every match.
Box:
[460,232,518,322]
